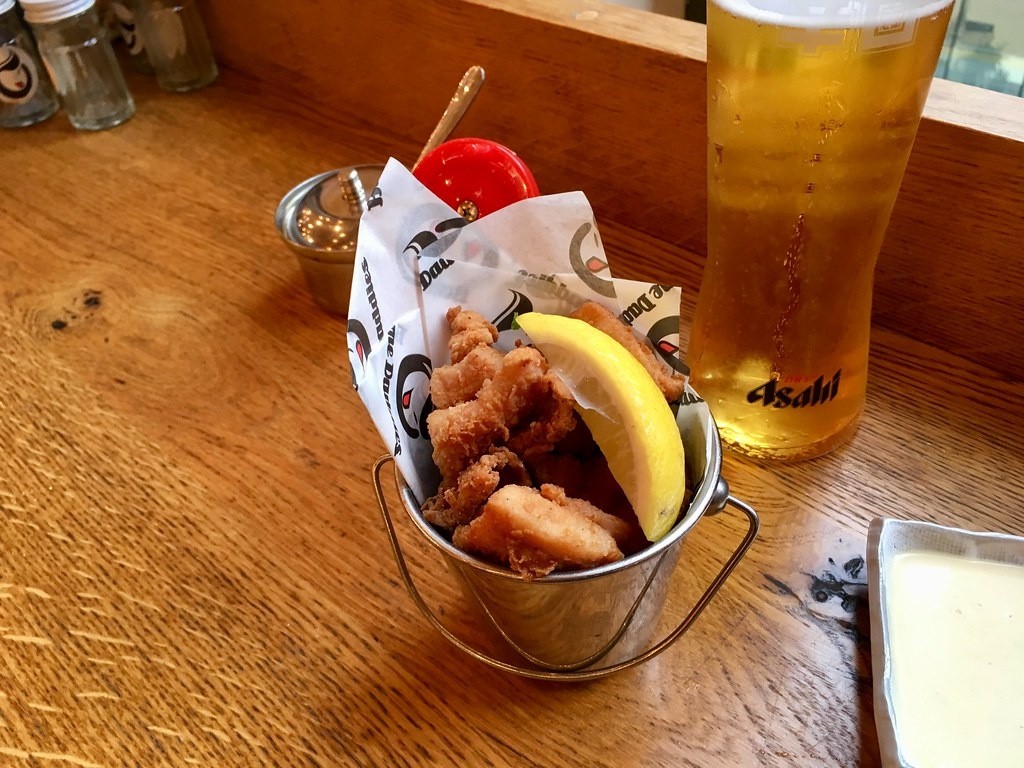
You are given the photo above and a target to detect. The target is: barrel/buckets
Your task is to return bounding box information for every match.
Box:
[370,355,760,682]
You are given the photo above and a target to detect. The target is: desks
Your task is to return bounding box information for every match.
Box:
[0,0,1024,768]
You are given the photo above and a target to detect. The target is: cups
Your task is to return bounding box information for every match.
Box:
[676,0,956,467]
[15,0,135,129]
[105,0,156,78]
[130,0,221,92]
[0,0,60,130]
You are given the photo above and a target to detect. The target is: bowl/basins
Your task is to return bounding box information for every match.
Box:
[272,163,418,319]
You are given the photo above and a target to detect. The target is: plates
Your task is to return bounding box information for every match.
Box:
[868,515,1024,768]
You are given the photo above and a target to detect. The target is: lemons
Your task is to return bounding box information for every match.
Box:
[516,313,685,542]
